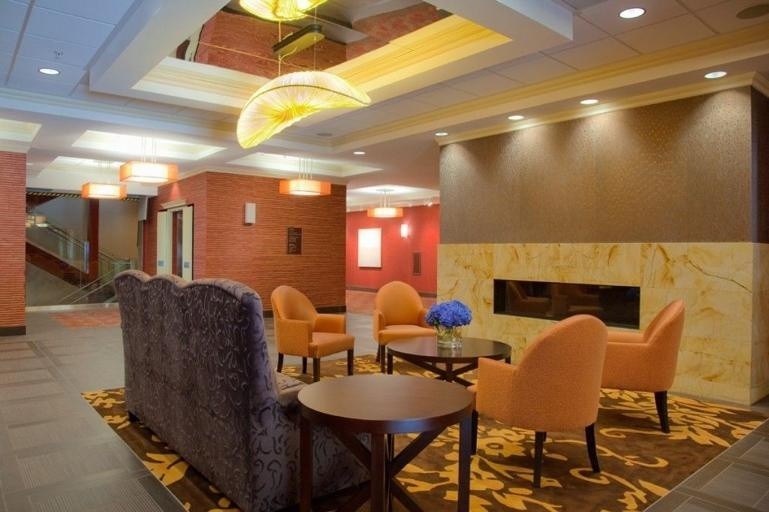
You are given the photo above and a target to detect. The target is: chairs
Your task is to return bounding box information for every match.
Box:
[267,281,439,382]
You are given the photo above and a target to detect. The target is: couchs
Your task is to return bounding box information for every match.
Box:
[113,269,393,511]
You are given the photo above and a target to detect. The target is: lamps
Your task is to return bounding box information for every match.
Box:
[78,132,179,201]
[239,0,327,41]
[234,29,404,220]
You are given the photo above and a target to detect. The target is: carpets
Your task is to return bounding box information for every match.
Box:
[78,355,769,512]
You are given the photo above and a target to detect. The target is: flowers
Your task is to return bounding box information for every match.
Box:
[425,299,474,338]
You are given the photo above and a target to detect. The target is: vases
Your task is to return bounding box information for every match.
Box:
[437,326,464,349]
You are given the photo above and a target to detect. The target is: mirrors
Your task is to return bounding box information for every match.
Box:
[171,0,459,89]
